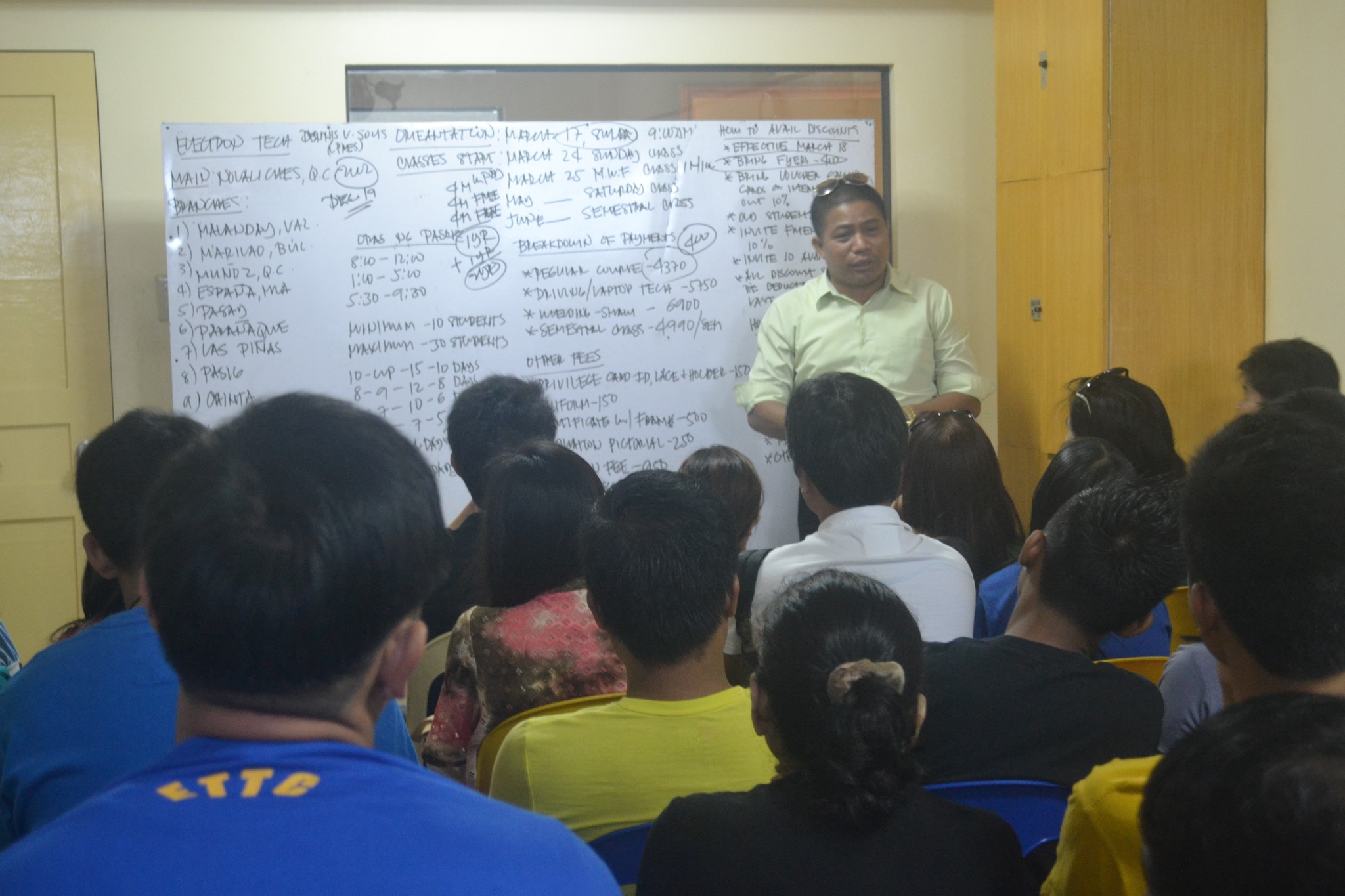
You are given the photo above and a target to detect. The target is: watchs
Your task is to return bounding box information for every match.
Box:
[903,407,916,428]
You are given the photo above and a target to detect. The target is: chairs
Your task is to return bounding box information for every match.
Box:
[401,585,1202,896]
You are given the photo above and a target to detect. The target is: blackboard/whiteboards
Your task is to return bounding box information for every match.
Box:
[162,118,876,550]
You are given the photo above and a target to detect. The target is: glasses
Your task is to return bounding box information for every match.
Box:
[811,173,870,198]
[909,410,974,434]
[1077,367,1129,406]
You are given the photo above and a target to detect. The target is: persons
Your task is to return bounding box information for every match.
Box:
[0,339,1345,896]
[736,178,990,541]
